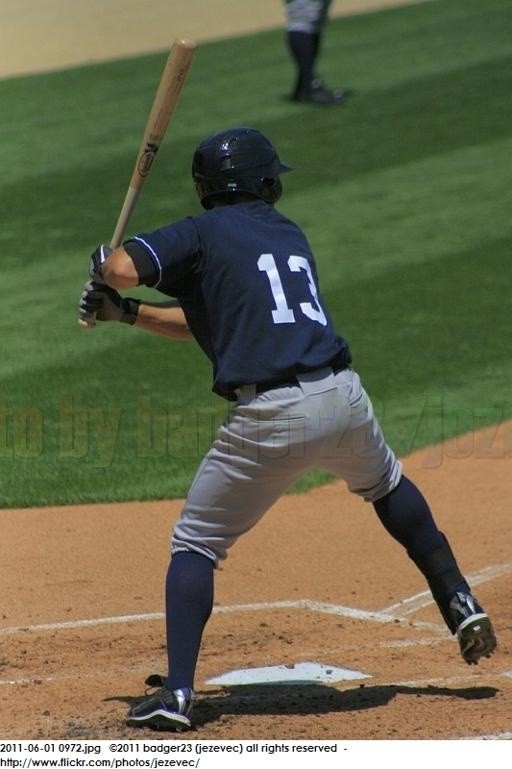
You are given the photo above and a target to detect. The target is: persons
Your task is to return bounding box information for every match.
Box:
[284,0,344,106]
[78,128,498,733]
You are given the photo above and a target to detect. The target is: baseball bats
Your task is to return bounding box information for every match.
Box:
[76,39,198,328]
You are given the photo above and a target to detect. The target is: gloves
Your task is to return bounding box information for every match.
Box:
[77,281,139,330]
[87,244,116,277]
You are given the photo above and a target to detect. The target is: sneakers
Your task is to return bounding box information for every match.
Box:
[447,593,497,668]
[122,685,197,734]
[285,75,339,108]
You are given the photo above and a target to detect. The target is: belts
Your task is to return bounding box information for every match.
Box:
[230,360,354,396]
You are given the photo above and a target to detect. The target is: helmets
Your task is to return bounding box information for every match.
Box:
[188,124,296,208]
